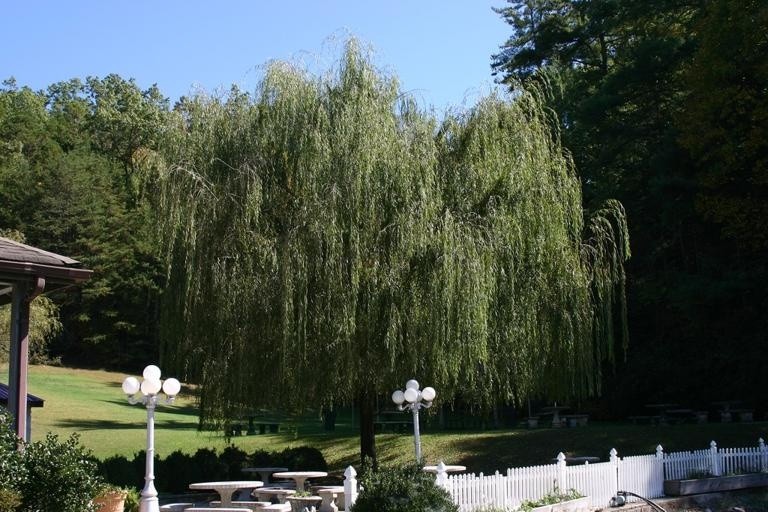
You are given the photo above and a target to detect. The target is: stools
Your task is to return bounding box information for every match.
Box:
[224,424,244,436]
[255,423,281,434]
[526,399,756,426]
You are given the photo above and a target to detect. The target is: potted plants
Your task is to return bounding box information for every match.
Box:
[93,483,139,511]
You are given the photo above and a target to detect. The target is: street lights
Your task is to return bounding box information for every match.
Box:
[122,366,181,512]
[392,380,436,468]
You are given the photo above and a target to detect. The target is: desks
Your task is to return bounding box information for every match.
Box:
[189,466,328,507]
[422,466,467,475]
[241,413,265,435]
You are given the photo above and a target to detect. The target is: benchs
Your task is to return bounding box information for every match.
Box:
[160,485,365,511]
